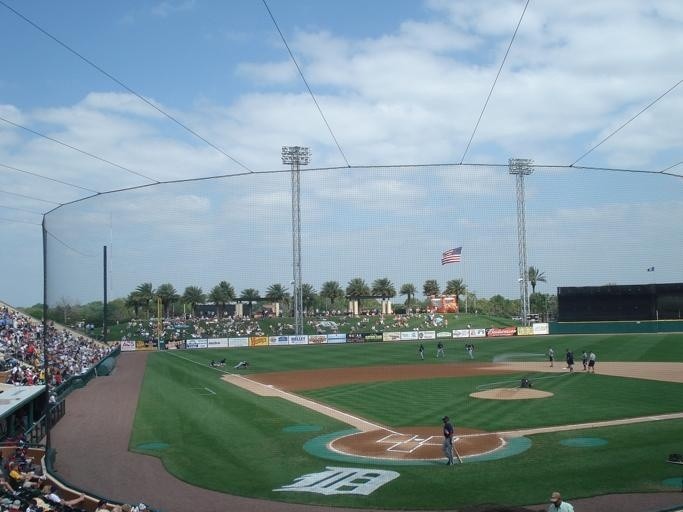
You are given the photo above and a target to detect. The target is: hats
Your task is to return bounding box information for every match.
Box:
[550,492,560,502]
[442,416,448,420]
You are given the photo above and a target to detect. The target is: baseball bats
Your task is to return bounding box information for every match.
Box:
[453,445,463,464]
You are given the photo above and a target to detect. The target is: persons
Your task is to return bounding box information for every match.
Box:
[311,313,447,334]
[131,314,264,340]
[548,347,554,367]
[588,351,595,373]
[569,351,574,372]
[416,344,424,360]
[582,351,587,370]
[442,416,454,465]
[521,377,533,387]
[436,342,445,358]
[211,358,248,368]
[567,349,570,368]
[0,432,149,512]
[548,492,574,512]
[0,304,118,403]
[465,345,474,359]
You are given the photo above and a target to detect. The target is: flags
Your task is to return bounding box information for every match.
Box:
[440,247,462,264]
[648,267,654,271]
